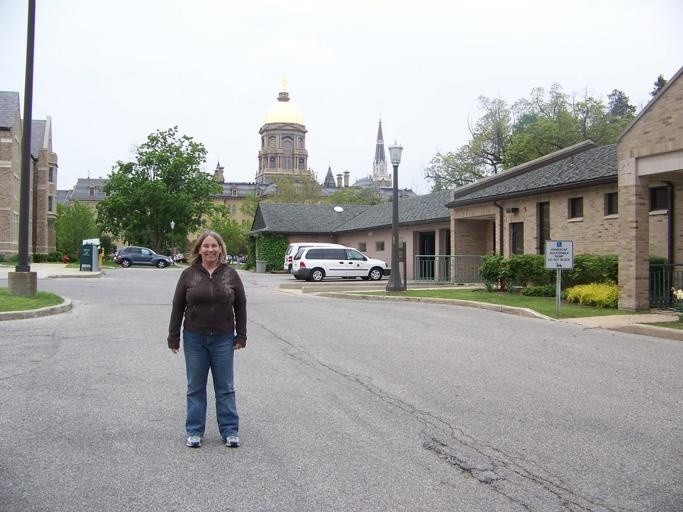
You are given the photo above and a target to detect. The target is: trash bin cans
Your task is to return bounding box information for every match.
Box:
[255,260,267,273]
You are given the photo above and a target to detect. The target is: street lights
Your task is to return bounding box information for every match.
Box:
[170,220,175,259]
[385,139,405,291]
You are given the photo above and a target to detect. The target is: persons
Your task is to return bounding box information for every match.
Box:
[62,253,71,264]
[165,230,249,449]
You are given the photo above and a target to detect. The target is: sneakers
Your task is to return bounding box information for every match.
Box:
[225,436,240,447]
[186,435,203,447]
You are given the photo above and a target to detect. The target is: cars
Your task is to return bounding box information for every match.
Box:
[226,255,246,263]
[173,254,185,263]
[112,246,173,268]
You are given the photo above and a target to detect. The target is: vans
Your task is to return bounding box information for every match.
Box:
[284,243,391,282]
